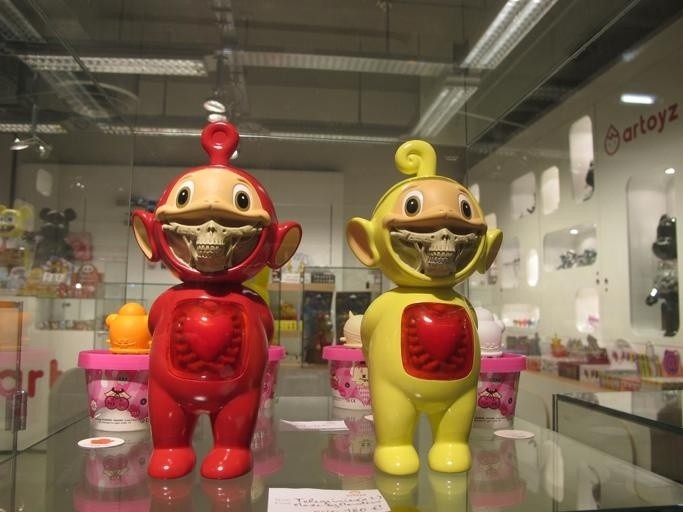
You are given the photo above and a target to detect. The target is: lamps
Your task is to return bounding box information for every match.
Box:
[9,71,52,158]
[202,0,230,123]
